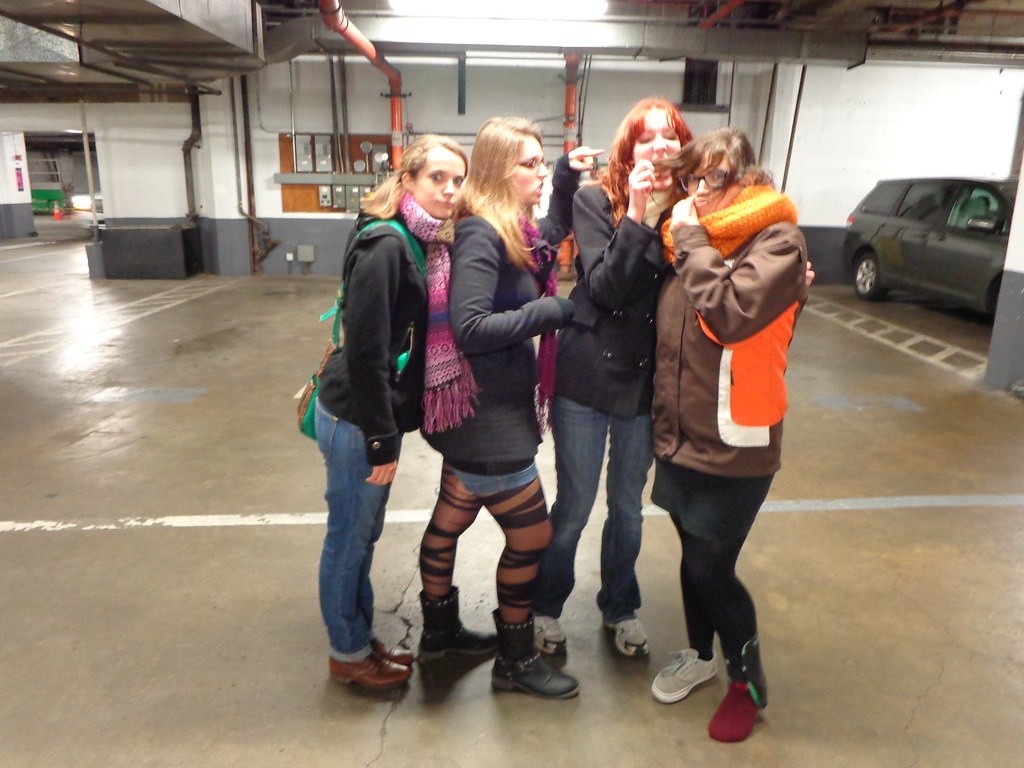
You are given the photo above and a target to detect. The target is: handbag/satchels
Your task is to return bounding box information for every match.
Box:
[298,347,411,441]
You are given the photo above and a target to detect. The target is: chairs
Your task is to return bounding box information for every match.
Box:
[957,198,996,234]
[901,194,942,224]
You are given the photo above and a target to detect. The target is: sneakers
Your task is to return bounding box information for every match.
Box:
[532,613,568,655]
[604,615,648,657]
[652,649,717,703]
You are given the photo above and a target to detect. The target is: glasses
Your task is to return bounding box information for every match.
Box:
[678,169,734,192]
[520,158,544,171]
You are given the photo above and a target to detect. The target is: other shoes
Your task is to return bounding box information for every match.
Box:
[710,683,760,741]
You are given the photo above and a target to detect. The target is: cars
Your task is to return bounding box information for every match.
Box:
[842,176,1019,318]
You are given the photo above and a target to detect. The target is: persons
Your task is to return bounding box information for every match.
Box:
[417,117,603,698]
[528,97,816,659]
[650,128,807,744]
[312,134,469,686]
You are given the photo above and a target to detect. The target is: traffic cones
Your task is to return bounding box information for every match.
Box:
[54,201,63,220]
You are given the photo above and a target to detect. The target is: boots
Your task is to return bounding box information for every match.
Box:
[492,604,580,699]
[418,585,497,660]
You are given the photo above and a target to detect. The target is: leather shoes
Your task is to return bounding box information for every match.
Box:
[330,638,414,685]
